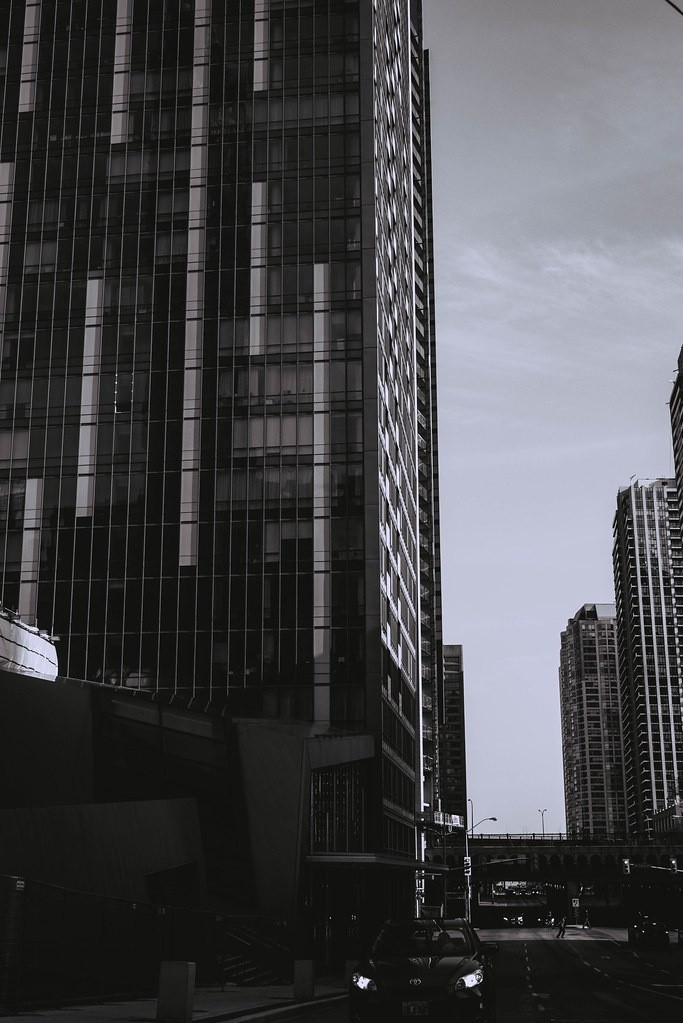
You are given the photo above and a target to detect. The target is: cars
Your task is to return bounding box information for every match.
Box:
[628,925,669,945]
[520,889,527,895]
[500,907,524,927]
[496,884,533,890]
[537,912,555,926]
[506,889,516,896]
[350,919,486,1020]
[531,889,539,895]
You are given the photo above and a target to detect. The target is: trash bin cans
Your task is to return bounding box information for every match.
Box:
[294,960,315,1002]
[157,960,195,1023]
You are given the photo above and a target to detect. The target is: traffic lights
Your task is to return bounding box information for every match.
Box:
[422,893,426,897]
[464,857,471,875]
[416,891,423,900]
[671,858,678,877]
[623,859,630,874]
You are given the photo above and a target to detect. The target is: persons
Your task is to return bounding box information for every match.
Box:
[545,911,555,928]
[556,916,566,938]
[438,933,462,955]
[582,909,592,929]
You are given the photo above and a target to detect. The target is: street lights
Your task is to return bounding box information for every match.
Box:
[464,817,497,922]
[538,808,548,836]
[468,798,474,839]
